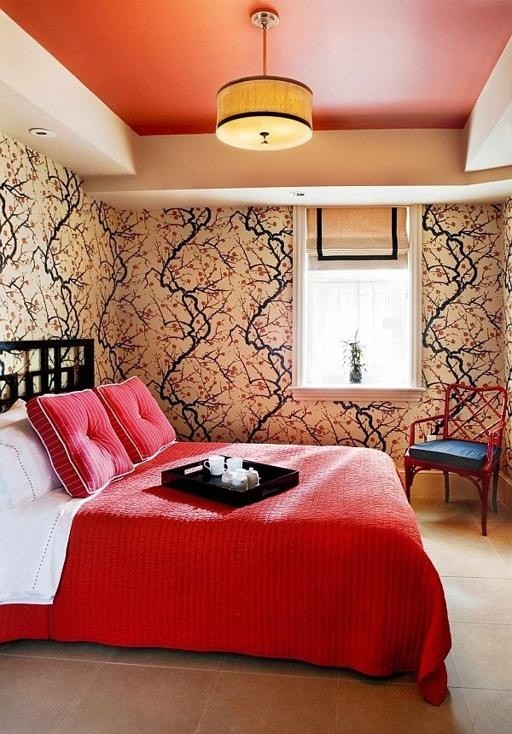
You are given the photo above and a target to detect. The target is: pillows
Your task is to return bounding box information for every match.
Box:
[0,375,177,510]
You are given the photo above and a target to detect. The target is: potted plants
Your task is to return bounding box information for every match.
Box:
[342,329,367,384]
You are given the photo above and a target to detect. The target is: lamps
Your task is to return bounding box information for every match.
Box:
[214,8,313,150]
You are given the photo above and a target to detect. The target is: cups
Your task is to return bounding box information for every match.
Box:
[204,456,259,490]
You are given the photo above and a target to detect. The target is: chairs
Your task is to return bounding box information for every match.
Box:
[403,382,509,537]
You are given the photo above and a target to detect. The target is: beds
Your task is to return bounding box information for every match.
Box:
[0,338,454,706]
